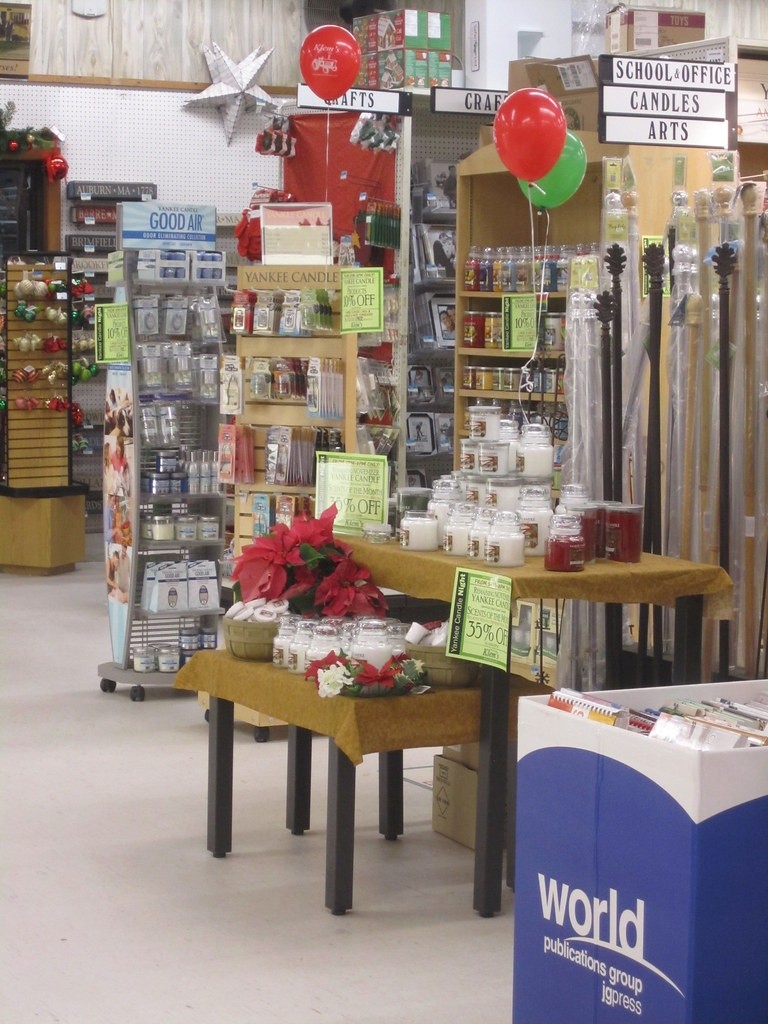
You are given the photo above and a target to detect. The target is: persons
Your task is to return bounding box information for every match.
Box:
[404,156,456,490]
[101,388,136,607]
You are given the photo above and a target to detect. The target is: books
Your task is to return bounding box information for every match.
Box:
[546,685,768,752]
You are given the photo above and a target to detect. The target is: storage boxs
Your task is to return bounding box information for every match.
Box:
[350,10,451,92]
[431,742,479,851]
[526,58,599,132]
[510,678,767,1022]
[604,4,705,54]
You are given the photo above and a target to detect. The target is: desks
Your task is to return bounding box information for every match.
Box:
[330,530,731,919]
[191,649,553,917]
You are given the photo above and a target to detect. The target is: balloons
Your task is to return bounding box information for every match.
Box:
[490,88,568,182]
[515,128,588,208]
[298,25,361,102]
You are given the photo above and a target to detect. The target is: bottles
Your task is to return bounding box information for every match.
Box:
[464,242,599,292]
[140,513,219,541]
[273,614,411,678]
[399,406,644,571]
[183,449,223,494]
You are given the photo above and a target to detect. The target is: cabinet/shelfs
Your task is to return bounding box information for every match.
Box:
[66,179,155,531]
[450,131,740,534]
[402,88,492,522]
[97,251,235,699]
[234,266,357,590]
